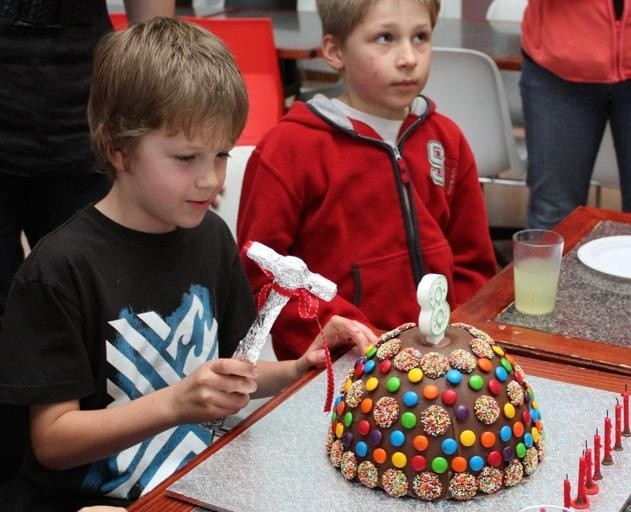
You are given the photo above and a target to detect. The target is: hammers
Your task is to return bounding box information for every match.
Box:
[200,241,338,431]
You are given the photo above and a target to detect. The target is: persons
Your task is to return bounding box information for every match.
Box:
[0,0,175,314]
[0,16,379,506]
[517,0,631,239]
[236,0,502,357]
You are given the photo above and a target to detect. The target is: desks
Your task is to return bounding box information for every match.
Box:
[188,8,524,70]
[125,204,631,512]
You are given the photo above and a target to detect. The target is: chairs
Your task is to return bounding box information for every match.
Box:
[296,1,339,75]
[105,12,286,146]
[486,1,526,130]
[418,45,530,184]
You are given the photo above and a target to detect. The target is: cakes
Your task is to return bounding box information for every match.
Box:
[324,272,546,504]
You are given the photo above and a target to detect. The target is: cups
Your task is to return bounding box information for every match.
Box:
[512,229,566,318]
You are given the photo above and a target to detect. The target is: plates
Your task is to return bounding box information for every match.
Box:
[576,234,631,284]
[572,262,630,296]
